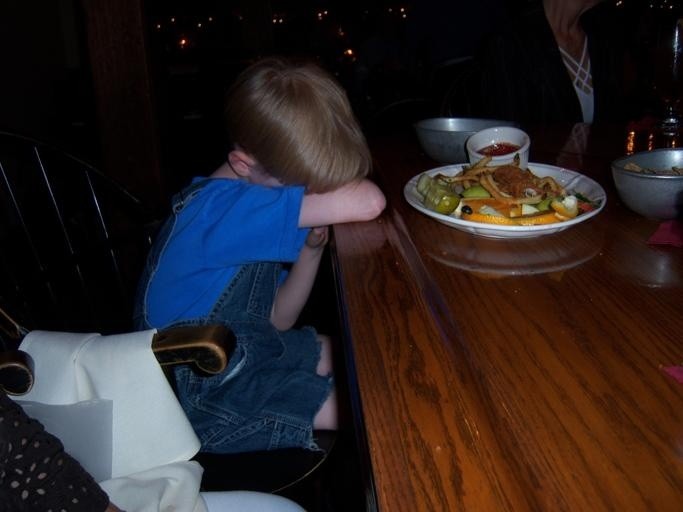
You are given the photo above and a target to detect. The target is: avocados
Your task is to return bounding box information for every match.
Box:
[423,184,461,216]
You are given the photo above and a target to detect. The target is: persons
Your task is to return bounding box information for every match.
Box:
[134,58,385,455]
[497,0,645,156]
[0,389,307,511]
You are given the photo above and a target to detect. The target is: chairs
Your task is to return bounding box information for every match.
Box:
[0,306,323,511]
[0,127,339,501]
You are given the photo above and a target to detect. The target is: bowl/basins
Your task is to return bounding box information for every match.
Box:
[611,146,682,222]
[411,115,514,162]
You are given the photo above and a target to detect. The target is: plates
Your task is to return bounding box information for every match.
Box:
[413,226,603,275]
[403,161,606,242]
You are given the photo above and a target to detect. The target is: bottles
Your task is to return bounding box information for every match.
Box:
[660,103,679,136]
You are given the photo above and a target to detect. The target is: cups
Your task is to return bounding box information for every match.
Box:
[464,125,530,174]
